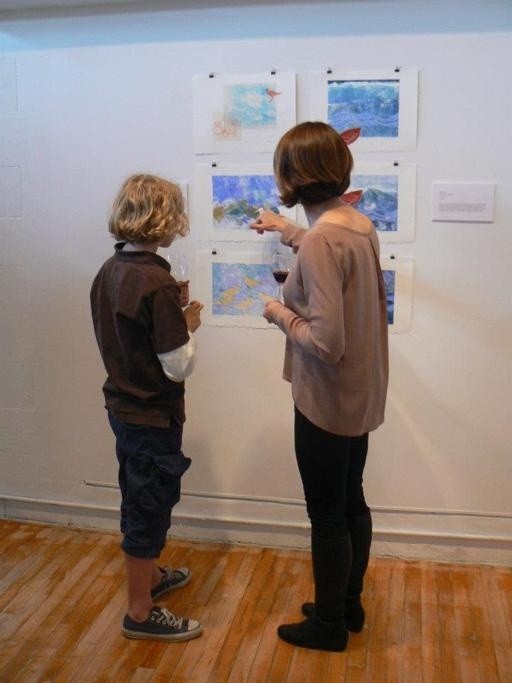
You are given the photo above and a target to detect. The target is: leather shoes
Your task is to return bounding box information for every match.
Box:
[301,600,366,633]
[275,610,351,653]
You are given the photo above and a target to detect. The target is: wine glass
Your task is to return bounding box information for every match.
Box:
[167,252,191,308]
[269,253,291,305]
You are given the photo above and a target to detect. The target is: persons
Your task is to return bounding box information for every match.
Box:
[250,121,389,653]
[89,172,204,643]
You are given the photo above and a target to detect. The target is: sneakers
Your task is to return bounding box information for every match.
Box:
[120,605,204,642]
[148,564,192,599]
